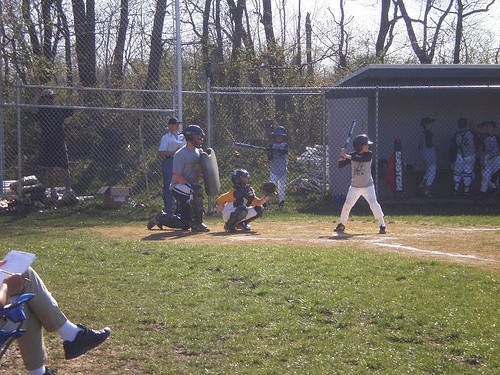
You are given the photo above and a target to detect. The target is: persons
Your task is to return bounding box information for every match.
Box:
[269,126,287,208]
[0,259,111,375]
[218,169,274,233]
[35,89,74,197]
[158,117,187,215]
[478,121,500,199]
[450,118,476,196]
[420,117,437,195]
[335,134,386,234]
[147,124,210,232]
[77,115,128,194]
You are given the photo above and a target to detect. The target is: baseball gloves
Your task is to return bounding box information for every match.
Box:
[261,182,276,197]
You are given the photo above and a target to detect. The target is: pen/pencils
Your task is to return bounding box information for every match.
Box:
[0,269,31,282]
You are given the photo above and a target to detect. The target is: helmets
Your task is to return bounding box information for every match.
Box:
[274,126,287,136]
[183,124,206,148]
[352,134,373,153]
[230,169,252,191]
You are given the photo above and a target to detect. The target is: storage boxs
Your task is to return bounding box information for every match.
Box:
[96,184,134,210]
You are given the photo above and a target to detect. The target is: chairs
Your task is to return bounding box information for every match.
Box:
[0,292,37,361]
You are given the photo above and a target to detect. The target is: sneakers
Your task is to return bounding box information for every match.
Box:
[379,225,386,234]
[424,191,435,199]
[191,222,211,232]
[333,222,346,232]
[278,200,285,207]
[147,210,166,229]
[452,189,458,195]
[480,191,486,200]
[63,323,111,360]
[417,183,426,191]
[488,186,497,194]
[236,223,251,232]
[224,222,237,234]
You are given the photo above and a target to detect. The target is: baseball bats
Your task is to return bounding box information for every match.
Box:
[233,141,270,150]
[341,120,358,159]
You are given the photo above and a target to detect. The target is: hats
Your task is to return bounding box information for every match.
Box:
[168,117,182,125]
[457,118,468,128]
[421,117,435,127]
[485,121,497,129]
